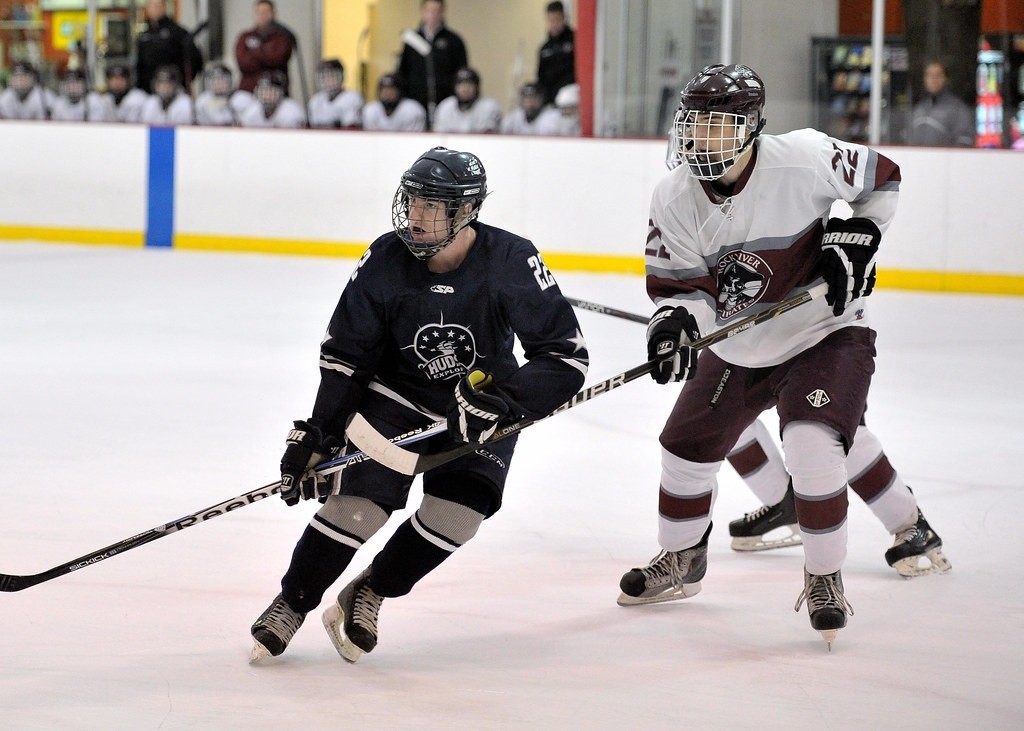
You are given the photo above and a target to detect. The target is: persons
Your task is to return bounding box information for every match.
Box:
[249,145,590,663]
[727,403,952,574]
[1,0,581,136]
[909,60,974,146]
[617,64,900,650]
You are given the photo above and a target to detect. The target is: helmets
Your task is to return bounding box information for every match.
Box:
[672,63,768,181]
[664,105,694,172]
[391,146,494,261]
[8,60,538,117]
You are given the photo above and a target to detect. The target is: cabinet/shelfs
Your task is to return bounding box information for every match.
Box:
[811,37,895,146]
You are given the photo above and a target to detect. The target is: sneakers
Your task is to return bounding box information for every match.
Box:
[249,590,306,664]
[617,521,713,606]
[794,563,854,652]
[885,486,953,580]
[321,564,384,663]
[728,475,802,551]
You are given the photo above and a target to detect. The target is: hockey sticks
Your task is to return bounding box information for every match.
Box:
[345,276,829,476]
[0,417,448,592]
[564,297,651,325]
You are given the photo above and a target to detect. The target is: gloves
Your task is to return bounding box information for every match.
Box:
[445,368,509,458]
[646,306,701,385]
[819,217,881,317]
[279,421,343,506]
